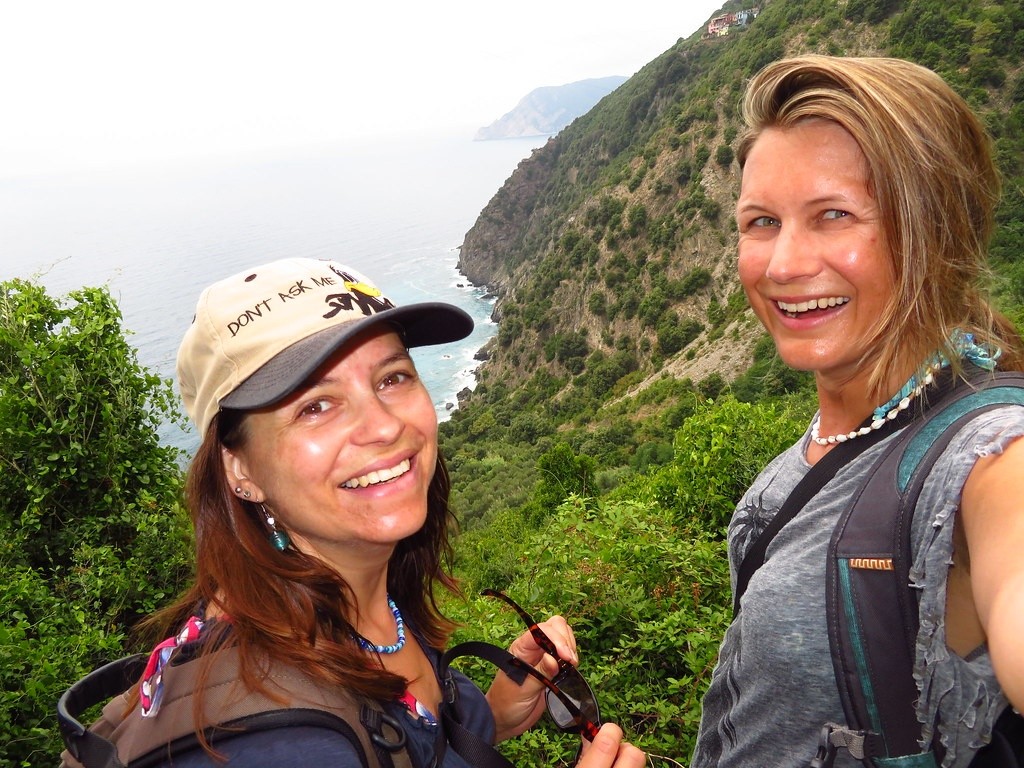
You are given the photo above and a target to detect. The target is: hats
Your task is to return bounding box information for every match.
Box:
[177,257,474,442]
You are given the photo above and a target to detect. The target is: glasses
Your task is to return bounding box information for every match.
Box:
[482,587,602,766]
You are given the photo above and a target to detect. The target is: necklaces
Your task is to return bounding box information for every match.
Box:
[811,363,940,446]
[352,593,405,652]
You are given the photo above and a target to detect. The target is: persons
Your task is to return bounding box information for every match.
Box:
[133,256,646,768]
[688,55,1023,768]
